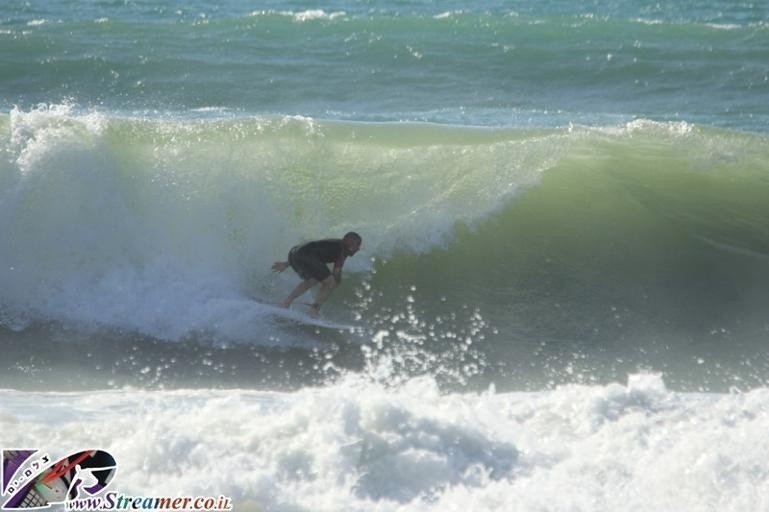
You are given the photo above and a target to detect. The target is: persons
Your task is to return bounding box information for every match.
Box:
[271,231,361,320]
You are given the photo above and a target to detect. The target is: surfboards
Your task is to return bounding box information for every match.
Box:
[247,298,367,331]
